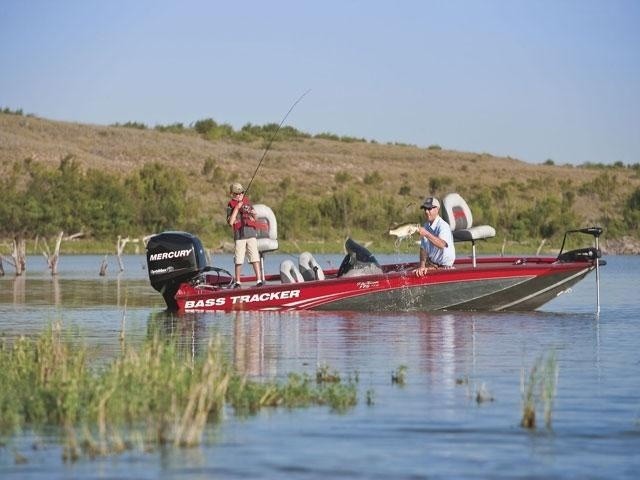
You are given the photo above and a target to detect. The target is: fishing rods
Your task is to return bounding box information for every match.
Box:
[242,89,311,202]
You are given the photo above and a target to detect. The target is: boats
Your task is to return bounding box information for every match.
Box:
[145,191,606,315]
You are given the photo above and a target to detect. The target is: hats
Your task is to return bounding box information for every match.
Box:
[420,197,440,209]
[230,182,245,195]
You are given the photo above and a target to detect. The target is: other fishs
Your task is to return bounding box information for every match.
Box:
[385,223,420,238]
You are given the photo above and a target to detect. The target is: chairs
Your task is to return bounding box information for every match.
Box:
[442,193,497,267]
[278,251,325,282]
[252,204,279,285]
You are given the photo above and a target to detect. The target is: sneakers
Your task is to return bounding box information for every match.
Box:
[233,281,241,289]
[256,279,263,287]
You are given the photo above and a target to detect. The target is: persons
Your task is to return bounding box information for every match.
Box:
[226,182,264,288]
[406,197,456,277]
